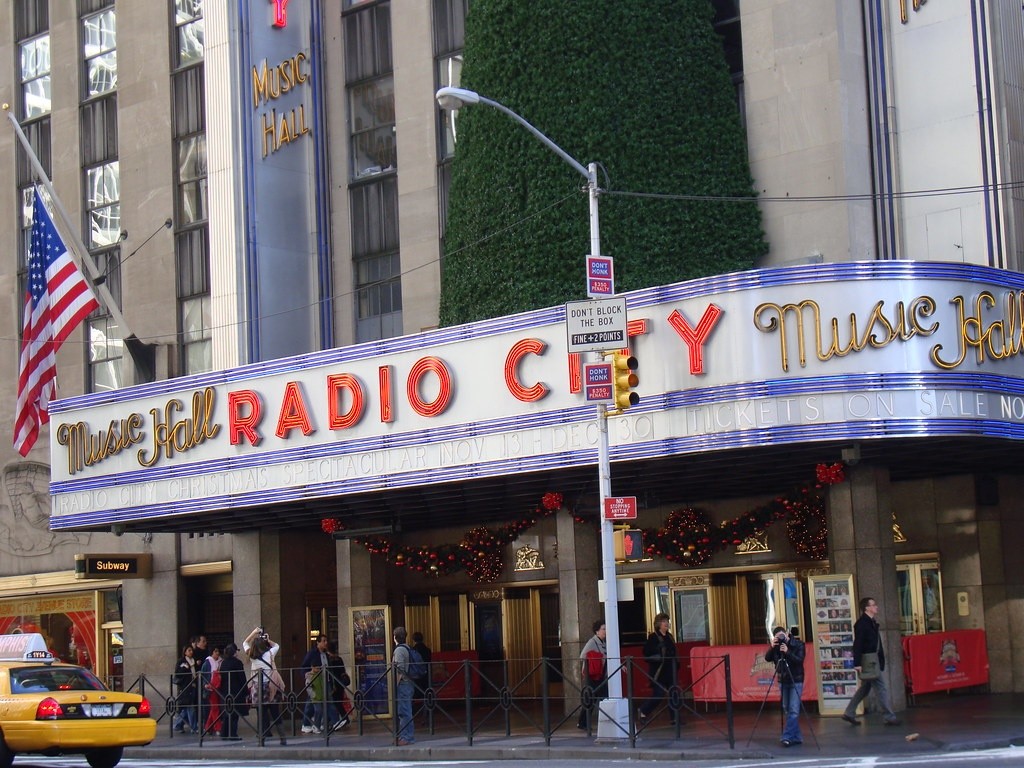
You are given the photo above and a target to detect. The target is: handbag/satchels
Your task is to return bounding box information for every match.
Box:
[173,658,185,685]
[202,690,210,701]
[267,650,285,691]
[860,653,881,680]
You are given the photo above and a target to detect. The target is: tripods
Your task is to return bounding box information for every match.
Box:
[747,644,821,751]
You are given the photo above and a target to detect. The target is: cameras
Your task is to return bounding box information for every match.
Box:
[258,626,267,640]
[775,635,787,644]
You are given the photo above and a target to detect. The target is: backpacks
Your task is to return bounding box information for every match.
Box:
[397,645,427,680]
[334,656,350,689]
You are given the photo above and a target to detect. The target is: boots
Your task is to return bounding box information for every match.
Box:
[277,724,286,745]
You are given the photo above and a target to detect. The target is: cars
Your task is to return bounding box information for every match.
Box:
[0,633,158,767]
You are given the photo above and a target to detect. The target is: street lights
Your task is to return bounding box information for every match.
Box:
[433,85,640,741]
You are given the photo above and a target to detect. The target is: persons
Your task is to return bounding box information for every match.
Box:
[172,635,250,740]
[241,625,289,746]
[391,628,417,746]
[298,634,351,734]
[814,587,854,695]
[577,620,628,731]
[636,613,687,726]
[841,597,904,726]
[409,630,432,696]
[764,627,807,746]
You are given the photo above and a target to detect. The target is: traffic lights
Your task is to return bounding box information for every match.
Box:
[614,352,640,412]
[613,530,643,565]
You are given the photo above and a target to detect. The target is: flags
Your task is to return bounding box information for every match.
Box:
[13,184,102,458]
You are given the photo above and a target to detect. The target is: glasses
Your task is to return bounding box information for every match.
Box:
[867,604,876,607]
[236,649,240,652]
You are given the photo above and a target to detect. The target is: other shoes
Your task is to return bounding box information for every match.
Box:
[886,719,902,726]
[392,737,413,746]
[793,736,803,743]
[173,724,240,741]
[301,717,349,734]
[781,738,792,746]
[637,708,647,725]
[842,714,861,725]
[576,723,592,731]
[671,718,686,725]
